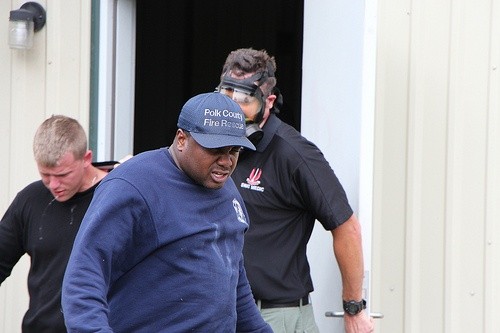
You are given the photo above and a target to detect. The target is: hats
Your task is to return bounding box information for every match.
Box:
[177,90,256,151]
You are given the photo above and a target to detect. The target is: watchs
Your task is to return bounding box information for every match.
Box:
[342,299,366,316]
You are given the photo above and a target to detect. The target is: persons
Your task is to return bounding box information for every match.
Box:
[217,48,376,333]
[60,92,275,333]
[0,115,108,333]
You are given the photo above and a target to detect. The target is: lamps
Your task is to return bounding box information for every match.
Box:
[7,1,46,49]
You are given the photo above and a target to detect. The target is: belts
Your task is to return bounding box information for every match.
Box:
[255,295,311,310]
